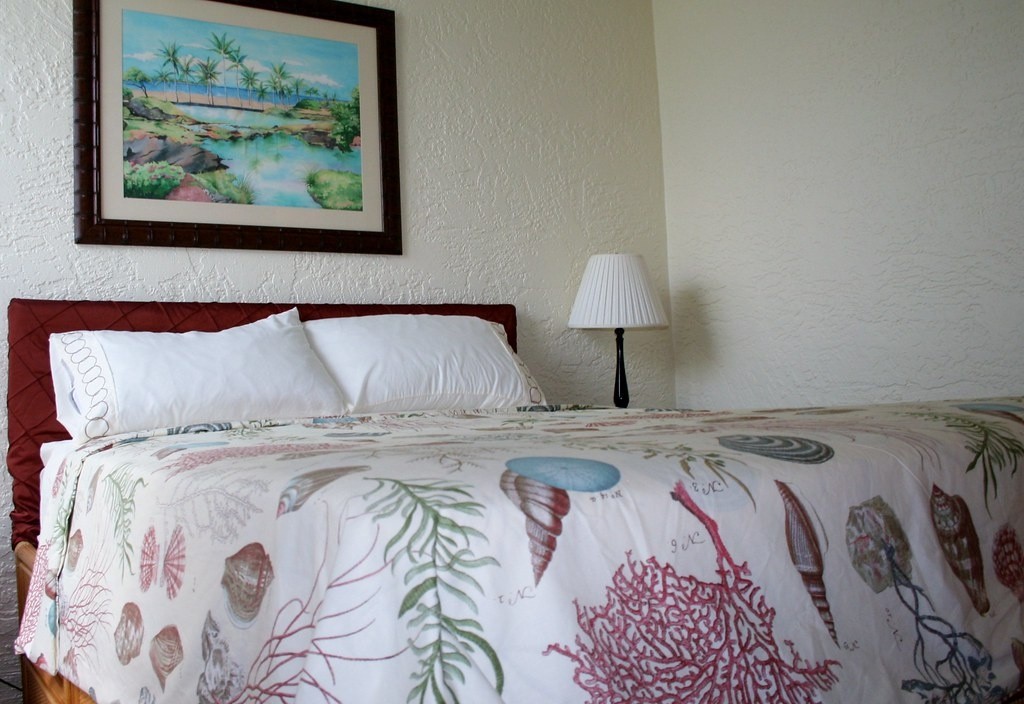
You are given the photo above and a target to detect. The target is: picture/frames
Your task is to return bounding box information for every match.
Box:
[72,0,403,256]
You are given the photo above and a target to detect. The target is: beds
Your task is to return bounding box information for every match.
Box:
[12,395,1024,704]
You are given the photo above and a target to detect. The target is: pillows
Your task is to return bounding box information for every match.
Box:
[48,307,351,443]
[302,314,548,414]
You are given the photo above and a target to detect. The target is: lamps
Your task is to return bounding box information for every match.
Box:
[567,253,670,408]
